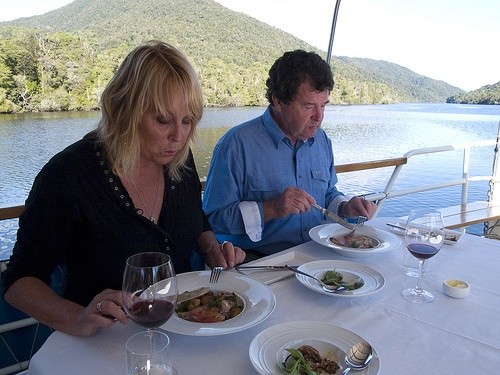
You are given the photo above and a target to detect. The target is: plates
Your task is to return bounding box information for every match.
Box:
[248,320,382,375]
[308,223,403,259]
[141,270,277,337]
[389,222,463,245]
[295,260,386,297]
[229,251,320,285]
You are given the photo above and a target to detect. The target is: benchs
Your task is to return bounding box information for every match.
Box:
[0,142,500,375]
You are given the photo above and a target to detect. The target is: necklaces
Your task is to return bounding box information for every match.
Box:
[130,168,161,224]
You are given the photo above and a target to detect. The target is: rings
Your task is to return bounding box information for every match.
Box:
[97,300,102,311]
[221,241,228,251]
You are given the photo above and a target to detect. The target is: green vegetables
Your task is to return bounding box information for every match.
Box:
[351,241,360,248]
[281,348,314,375]
[321,271,364,290]
[180,291,243,312]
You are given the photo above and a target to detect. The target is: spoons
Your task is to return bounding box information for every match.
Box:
[336,341,374,375]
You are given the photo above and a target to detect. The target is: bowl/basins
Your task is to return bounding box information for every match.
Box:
[443,279,471,299]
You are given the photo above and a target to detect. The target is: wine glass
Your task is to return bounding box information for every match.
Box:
[121,252,179,375]
[401,209,445,304]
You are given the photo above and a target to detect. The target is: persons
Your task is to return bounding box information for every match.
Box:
[0,40,246,338]
[203,49,378,267]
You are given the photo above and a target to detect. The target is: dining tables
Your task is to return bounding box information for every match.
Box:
[32,216,500,375]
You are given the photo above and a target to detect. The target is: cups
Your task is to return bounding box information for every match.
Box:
[125,330,178,375]
[402,248,428,278]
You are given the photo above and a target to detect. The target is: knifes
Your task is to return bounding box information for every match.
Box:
[166,287,210,305]
[310,203,357,231]
[284,264,347,293]
[235,265,299,271]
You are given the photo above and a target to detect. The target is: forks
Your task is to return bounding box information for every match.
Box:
[358,216,366,228]
[208,266,224,284]
